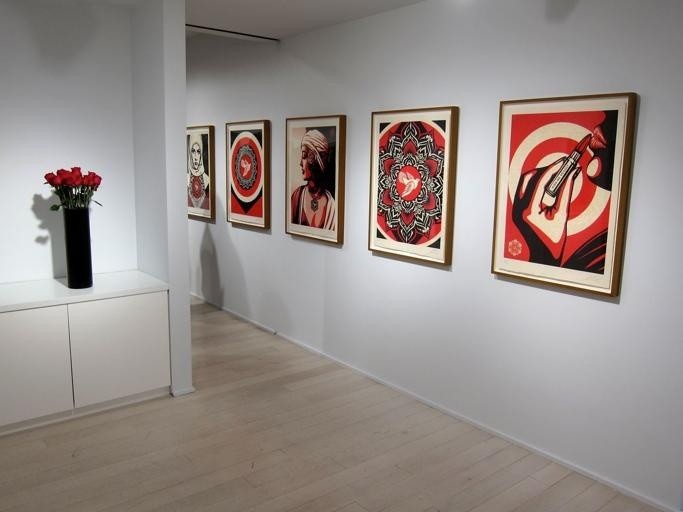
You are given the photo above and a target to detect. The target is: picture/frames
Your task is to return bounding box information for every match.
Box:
[184,120,273,232]
[280,114,347,246]
[488,89,638,301]
[362,104,460,267]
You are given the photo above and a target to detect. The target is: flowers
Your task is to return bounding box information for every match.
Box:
[46,165,103,212]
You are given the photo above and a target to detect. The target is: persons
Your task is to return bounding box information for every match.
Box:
[289,129,335,230]
[187,134,209,209]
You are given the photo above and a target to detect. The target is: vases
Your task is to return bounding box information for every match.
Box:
[61,209,94,290]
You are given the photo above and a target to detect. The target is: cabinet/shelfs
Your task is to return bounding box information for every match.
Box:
[0,267,170,438]
